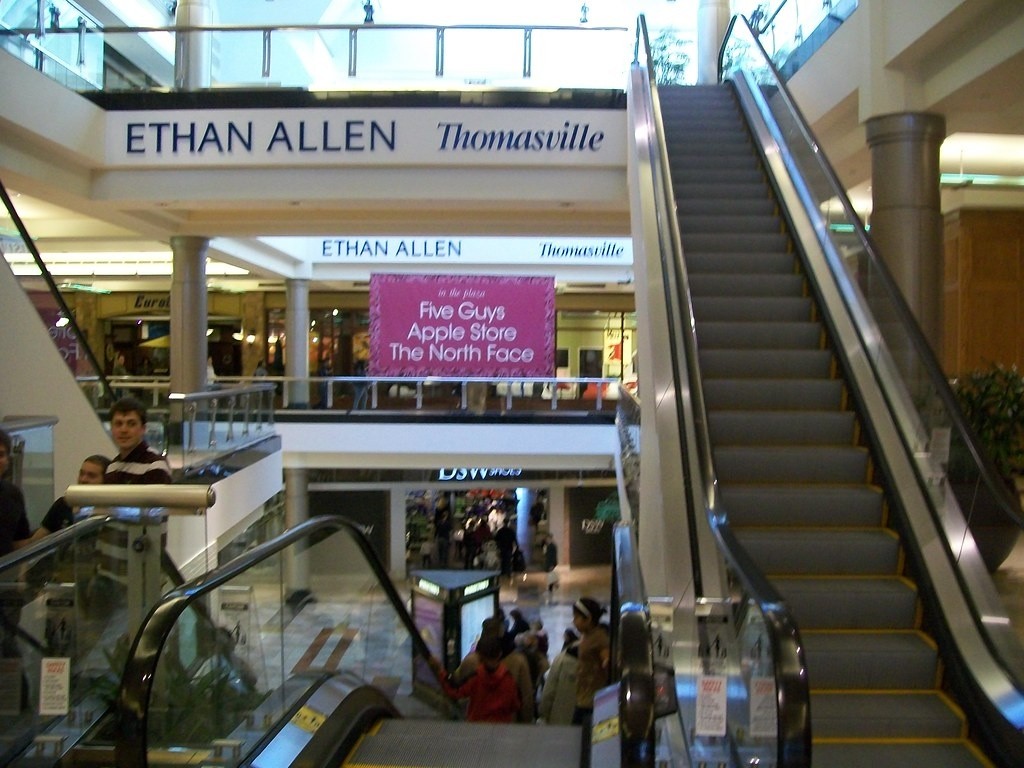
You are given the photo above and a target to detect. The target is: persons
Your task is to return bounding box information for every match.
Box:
[530,382,543,400]
[313,358,335,409]
[207,356,219,407]
[541,534,560,599]
[419,494,524,586]
[254,361,270,409]
[530,496,543,533]
[112,355,134,399]
[450,382,462,409]
[0,401,172,701]
[352,360,369,410]
[449,610,550,724]
[541,598,616,724]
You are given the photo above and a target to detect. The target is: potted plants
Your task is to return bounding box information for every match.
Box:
[912,358,1024,571]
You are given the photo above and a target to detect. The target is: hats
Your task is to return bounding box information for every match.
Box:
[481,618,505,640]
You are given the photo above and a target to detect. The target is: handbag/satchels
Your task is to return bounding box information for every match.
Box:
[512,549,526,573]
